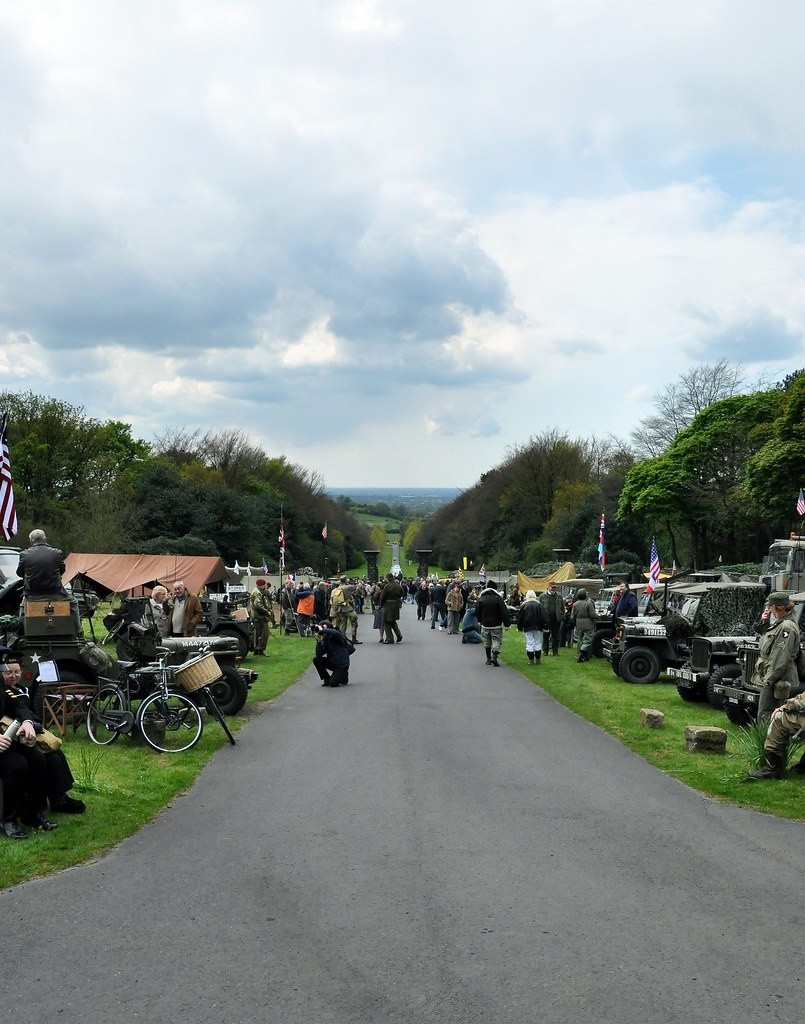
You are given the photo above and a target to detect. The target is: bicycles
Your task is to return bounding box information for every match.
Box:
[87,645,203,754]
[166,643,238,746]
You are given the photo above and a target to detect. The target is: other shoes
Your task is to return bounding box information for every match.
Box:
[322,676,330,686]
[383,640,395,644]
[577,650,590,662]
[49,794,87,813]
[438,625,443,630]
[396,636,403,642]
[747,747,785,779]
[379,639,383,643]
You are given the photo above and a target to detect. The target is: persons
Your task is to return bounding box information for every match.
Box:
[163,581,203,664]
[0,645,86,839]
[250,565,639,687]
[16,529,84,638]
[748,691,805,779]
[141,586,169,639]
[753,593,800,725]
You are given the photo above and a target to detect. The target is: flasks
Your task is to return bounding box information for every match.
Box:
[0,719,21,752]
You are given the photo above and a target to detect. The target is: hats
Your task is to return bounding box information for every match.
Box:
[487,580,497,590]
[549,581,557,587]
[578,588,586,594]
[256,579,266,586]
[0,645,25,671]
[764,592,790,605]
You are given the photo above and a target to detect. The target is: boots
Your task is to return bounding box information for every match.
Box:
[527,650,541,665]
[485,646,492,665]
[491,650,500,667]
[351,635,362,644]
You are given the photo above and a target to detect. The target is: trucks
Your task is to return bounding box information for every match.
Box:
[759,532,805,598]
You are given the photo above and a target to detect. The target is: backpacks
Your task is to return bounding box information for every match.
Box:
[329,585,345,605]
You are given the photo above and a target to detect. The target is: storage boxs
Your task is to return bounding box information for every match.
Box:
[26,595,78,637]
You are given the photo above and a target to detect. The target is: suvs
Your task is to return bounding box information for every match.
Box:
[555,572,766,686]
[667,592,805,725]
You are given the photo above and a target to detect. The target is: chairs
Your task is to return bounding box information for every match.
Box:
[35,661,100,738]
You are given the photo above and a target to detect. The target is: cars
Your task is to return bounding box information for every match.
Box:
[0,577,257,718]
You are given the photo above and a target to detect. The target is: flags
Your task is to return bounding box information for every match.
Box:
[278,518,285,570]
[234,560,239,575]
[247,562,251,577]
[0,409,18,543]
[459,567,465,580]
[672,560,677,575]
[797,489,805,516]
[479,564,486,582]
[264,565,268,575]
[322,521,327,538]
[646,538,661,594]
[598,509,605,572]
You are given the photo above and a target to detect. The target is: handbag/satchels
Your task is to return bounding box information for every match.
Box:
[0,716,61,754]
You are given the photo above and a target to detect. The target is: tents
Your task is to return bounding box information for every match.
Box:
[60,553,230,599]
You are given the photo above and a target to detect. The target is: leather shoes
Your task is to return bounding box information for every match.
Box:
[0,813,58,839]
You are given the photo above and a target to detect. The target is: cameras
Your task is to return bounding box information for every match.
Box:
[315,626,319,633]
